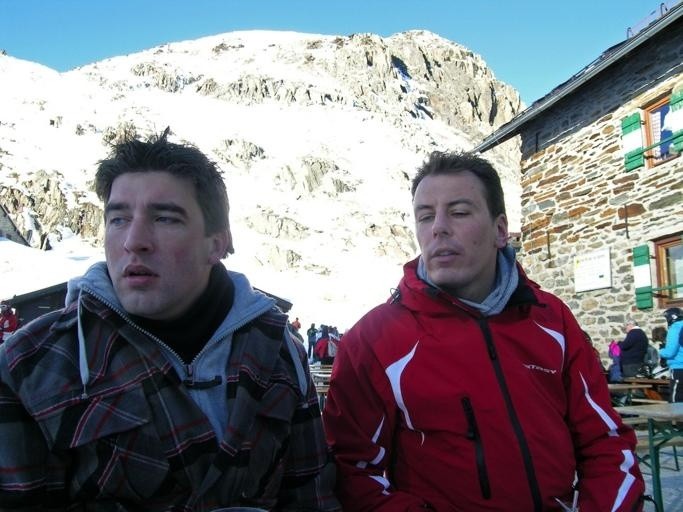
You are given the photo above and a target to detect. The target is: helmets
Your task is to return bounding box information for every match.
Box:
[667,308,683,326]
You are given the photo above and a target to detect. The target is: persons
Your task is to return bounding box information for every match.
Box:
[583,308,682,432]
[305,322,339,363]
[0,127,341,510]
[317,148,649,512]
[0,299,16,343]
[289,317,301,333]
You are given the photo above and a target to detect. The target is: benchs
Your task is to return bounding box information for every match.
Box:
[604,376,682,512]
[304,364,333,408]
